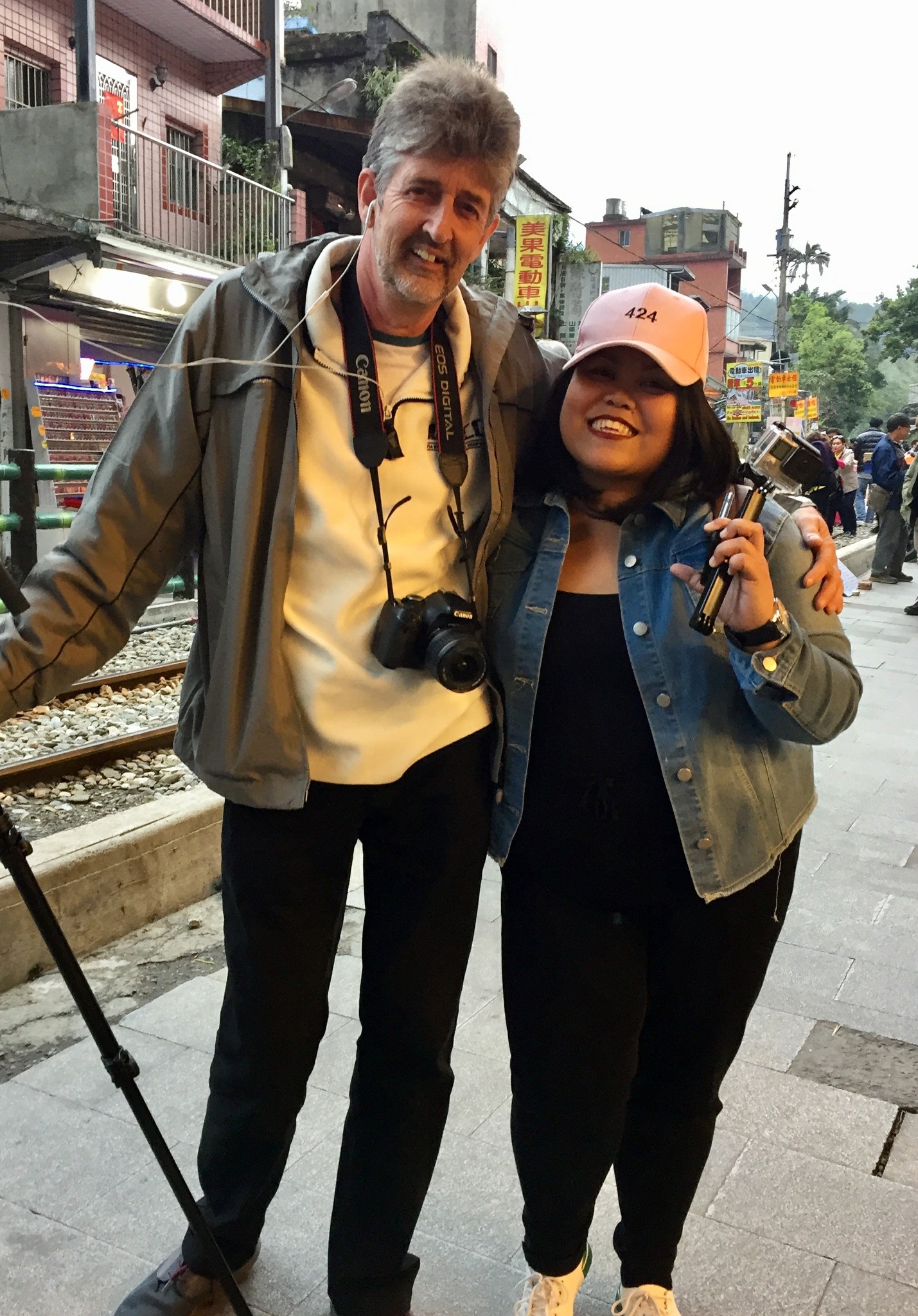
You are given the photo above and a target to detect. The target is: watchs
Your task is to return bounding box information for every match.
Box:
[722,597,793,648]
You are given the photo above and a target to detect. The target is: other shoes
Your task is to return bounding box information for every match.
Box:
[868,573,898,584]
[840,530,857,536]
[866,520,878,527]
[904,601,918,616]
[903,548,918,562]
[856,520,866,527]
[889,572,913,582]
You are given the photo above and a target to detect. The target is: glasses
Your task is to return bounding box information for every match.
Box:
[833,435,845,440]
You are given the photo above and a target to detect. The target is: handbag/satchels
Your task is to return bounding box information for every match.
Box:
[865,483,891,516]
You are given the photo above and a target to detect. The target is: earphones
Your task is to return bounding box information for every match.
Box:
[365,198,378,225]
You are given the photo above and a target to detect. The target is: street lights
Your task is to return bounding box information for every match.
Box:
[762,284,786,375]
[268,78,357,246]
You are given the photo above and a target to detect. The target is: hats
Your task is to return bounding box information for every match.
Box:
[562,282,709,390]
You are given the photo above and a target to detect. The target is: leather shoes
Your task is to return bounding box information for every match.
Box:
[113,1236,262,1316]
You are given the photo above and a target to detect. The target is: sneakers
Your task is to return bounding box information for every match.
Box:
[513,1242,592,1316]
[611,1283,680,1316]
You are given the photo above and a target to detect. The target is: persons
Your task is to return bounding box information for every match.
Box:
[869,413,913,584]
[896,436,918,563]
[853,417,888,526]
[484,282,866,1316]
[848,437,857,449]
[826,427,842,445]
[0,54,847,1315]
[831,435,860,538]
[802,431,839,537]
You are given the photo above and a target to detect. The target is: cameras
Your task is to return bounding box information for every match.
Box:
[369,591,490,692]
[737,422,824,495]
